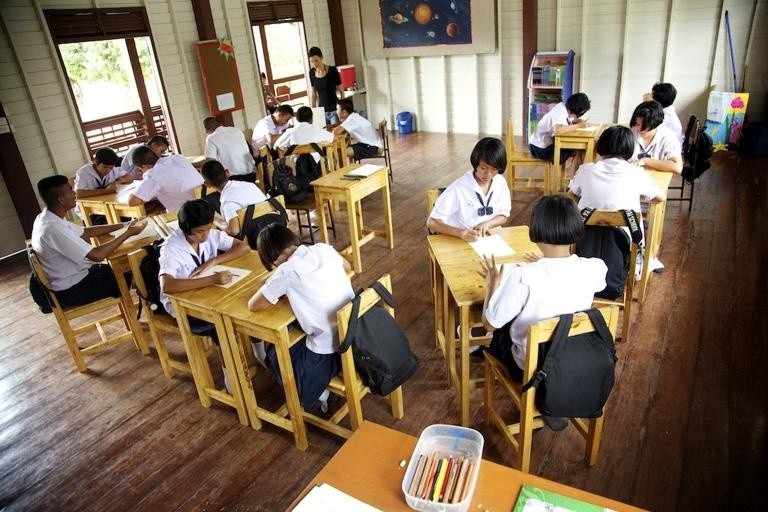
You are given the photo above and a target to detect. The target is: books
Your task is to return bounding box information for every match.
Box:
[291,482,384,512]
[512,483,620,511]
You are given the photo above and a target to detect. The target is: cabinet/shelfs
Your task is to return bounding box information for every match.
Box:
[527,49,575,144]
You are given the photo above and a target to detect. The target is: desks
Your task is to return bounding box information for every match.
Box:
[310,163,394,274]
[163,249,274,426]
[89,212,179,356]
[74,155,215,228]
[637,166,673,304]
[284,419,649,512]
[213,268,355,452]
[426,225,544,428]
[552,121,605,199]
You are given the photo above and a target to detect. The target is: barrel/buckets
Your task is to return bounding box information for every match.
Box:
[336,64,356,90]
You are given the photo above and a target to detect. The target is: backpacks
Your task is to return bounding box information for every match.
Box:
[575,206,644,300]
[521,307,618,419]
[338,281,421,399]
[271,158,308,203]
[28,247,57,315]
[241,197,291,251]
[295,152,323,194]
[136,238,165,320]
[681,115,714,182]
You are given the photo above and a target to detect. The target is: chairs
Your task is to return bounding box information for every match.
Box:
[667,115,701,212]
[254,132,353,246]
[127,243,255,379]
[25,238,151,373]
[506,112,551,196]
[579,209,642,344]
[425,188,447,304]
[236,195,286,249]
[354,118,393,192]
[300,271,404,439]
[483,304,619,474]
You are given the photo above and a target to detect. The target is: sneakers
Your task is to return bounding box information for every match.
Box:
[653,257,666,275]
[320,389,339,415]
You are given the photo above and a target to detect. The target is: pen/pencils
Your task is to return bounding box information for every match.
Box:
[211,271,241,277]
[340,174,368,181]
[119,186,136,196]
[452,213,483,241]
[407,451,476,506]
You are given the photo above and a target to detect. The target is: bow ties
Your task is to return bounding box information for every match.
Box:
[474,190,495,217]
[96,178,107,190]
